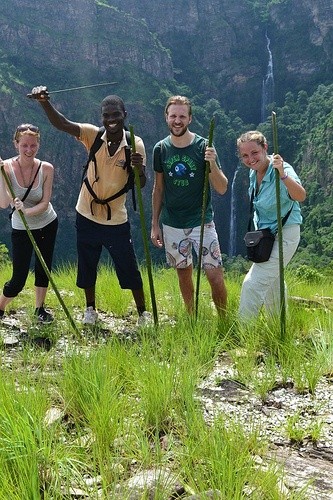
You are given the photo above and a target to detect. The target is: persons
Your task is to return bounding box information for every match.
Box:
[151,96,228,315]
[32,85,150,327]
[236,130,306,324]
[0,123,59,324]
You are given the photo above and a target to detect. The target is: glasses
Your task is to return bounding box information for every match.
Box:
[19,125,38,133]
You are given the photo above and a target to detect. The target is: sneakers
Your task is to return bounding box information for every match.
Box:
[34,307,54,324]
[139,311,154,325]
[82,306,98,326]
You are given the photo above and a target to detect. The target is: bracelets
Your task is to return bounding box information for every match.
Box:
[281,172,288,180]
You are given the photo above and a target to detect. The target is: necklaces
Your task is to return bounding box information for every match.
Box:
[108,138,121,145]
[19,162,35,187]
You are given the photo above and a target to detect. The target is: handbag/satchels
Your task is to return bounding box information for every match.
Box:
[244,227,275,262]
